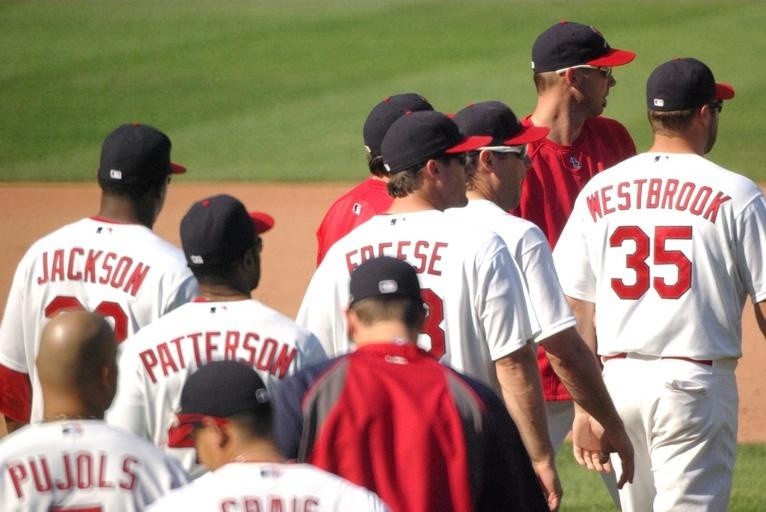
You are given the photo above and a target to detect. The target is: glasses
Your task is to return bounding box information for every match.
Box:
[703,98,724,109]
[554,63,614,75]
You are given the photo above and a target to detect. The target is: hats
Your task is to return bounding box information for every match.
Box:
[168,361,269,447]
[100,124,185,183]
[364,93,550,173]
[532,20,635,74]
[350,258,419,300]
[180,196,274,266]
[647,58,734,111]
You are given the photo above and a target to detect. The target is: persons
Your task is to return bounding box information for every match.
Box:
[296,111,564,512]
[103,194,330,482]
[551,55,765,511]
[502,19,636,510]
[136,360,392,511]
[0,311,189,511]
[0,120,200,436]
[268,255,551,511]
[296,93,635,490]
